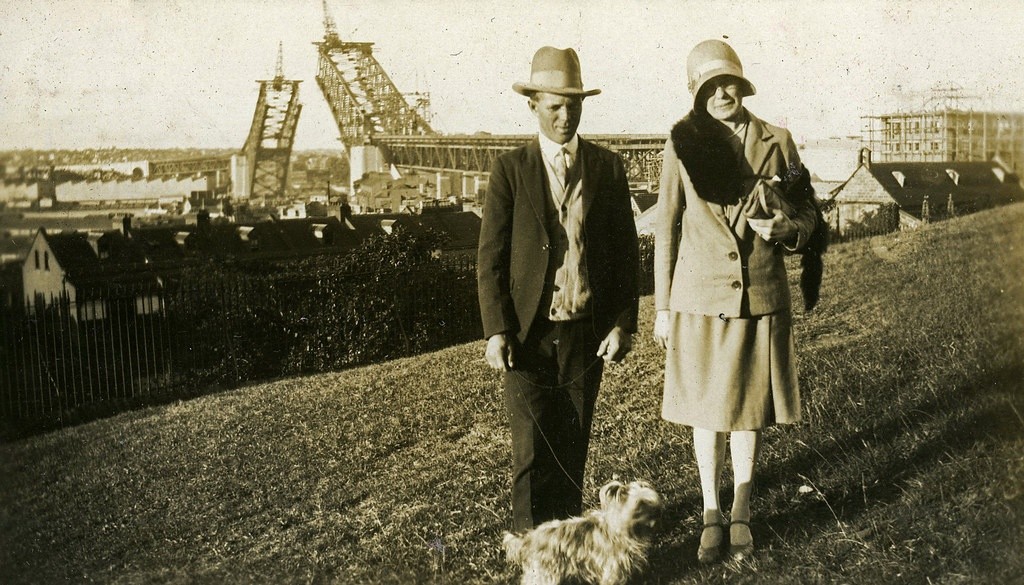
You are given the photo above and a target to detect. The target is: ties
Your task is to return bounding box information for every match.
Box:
[557,148,567,192]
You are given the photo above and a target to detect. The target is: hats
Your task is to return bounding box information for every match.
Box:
[686,39,757,116]
[512,46,601,96]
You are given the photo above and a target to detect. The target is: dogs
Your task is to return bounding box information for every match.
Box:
[503,481,664,585]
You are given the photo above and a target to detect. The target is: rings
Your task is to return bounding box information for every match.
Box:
[757,231,763,237]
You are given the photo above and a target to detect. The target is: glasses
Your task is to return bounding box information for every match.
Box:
[701,78,733,96]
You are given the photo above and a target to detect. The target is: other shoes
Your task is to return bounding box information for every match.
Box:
[697,523,726,565]
[726,513,754,560]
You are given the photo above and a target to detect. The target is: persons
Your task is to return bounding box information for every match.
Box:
[653,39,817,566]
[475,45,641,530]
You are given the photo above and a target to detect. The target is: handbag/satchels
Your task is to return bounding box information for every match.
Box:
[744,178,797,222]
